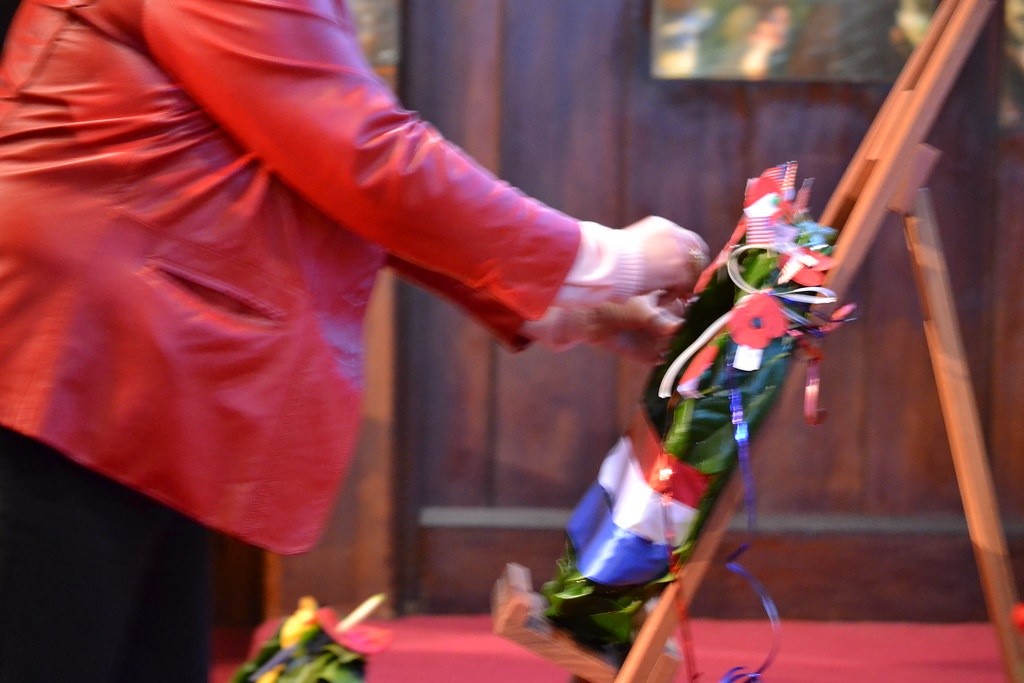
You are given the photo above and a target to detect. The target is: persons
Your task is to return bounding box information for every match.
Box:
[1,0,712,683]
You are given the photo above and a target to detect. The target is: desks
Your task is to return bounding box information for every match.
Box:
[250,616,1024,683]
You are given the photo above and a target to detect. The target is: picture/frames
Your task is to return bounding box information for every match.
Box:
[635,0,989,92]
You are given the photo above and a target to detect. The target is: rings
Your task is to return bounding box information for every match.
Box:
[688,247,705,271]
